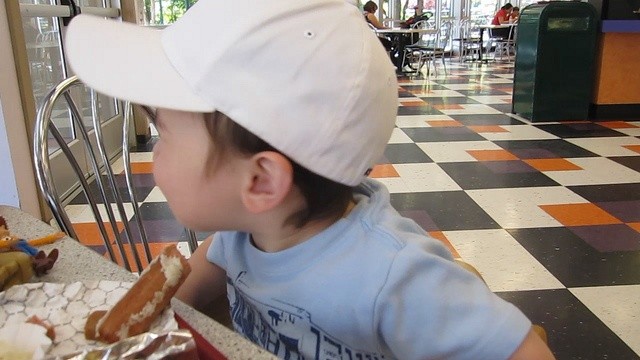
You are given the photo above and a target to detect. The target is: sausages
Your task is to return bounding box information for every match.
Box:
[95,243,192,346]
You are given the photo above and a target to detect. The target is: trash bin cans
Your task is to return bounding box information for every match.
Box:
[511,1,598,123]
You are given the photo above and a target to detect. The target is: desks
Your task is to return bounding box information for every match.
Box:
[26,41,59,87]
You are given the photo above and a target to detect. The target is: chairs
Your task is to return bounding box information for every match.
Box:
[417,22,451,78]
[492,20,518,63]
[485,15,504,60]
[33,75,198,277]
[401,21,431,76]
[459,19,488,68]
[30,31,59,84]
[368,23,377,36]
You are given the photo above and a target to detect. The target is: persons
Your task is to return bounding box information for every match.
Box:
[507,7,520,22]
[362,0,390,30]
[492,3,513,54]
[64,0,558,360]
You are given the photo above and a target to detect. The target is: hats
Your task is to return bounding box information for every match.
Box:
[64,1,399,187]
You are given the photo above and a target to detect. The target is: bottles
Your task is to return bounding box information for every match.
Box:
[509,16,513,24]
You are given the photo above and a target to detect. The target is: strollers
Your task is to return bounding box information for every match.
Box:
[387,11,433,71]
